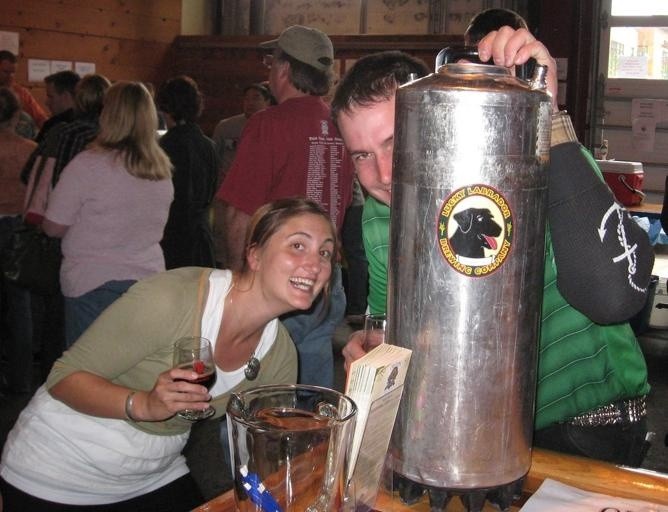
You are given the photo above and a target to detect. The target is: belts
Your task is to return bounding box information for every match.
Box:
[564,394,647,428]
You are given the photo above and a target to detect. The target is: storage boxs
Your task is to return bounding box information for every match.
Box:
[594,160,646,206]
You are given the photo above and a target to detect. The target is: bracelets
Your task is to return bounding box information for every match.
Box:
[551,109,568,116]
[126,392,135,420]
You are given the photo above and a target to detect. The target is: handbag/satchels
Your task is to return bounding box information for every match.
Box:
[2,216,60,295]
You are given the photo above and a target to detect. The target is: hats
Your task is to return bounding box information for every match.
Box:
[259,25,335,71]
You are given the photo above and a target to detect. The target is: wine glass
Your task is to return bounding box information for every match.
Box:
[171,337,216,420]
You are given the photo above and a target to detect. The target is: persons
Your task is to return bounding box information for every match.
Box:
[1,196,336,511]
[331,28,658,468]
[453,9,529,64]
[210,23,356,392]
[0,51,271,395]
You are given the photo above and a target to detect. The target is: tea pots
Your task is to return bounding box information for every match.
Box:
[226,381,361,511]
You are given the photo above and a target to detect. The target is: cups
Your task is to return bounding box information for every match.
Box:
[362,313,386,352]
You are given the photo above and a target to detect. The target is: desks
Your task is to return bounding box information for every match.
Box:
[188,419,666,509]
[620,202,661,222]
